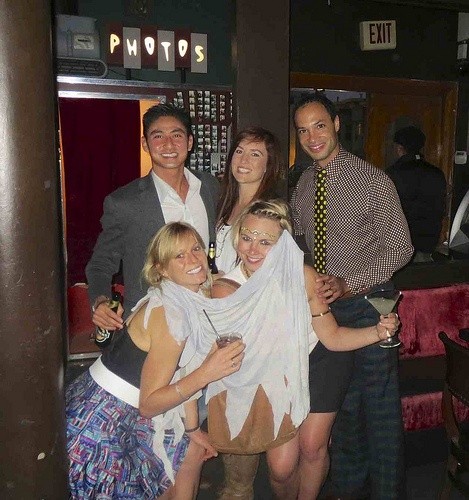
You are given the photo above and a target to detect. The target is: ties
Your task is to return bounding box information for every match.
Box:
[313,168,327,274]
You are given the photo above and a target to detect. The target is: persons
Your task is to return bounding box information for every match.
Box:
[383,126,447,255]
[285,94,415,500]
[85,104,222,428]
[211,196,402,500]
[209,124,286,500]
[65,222,247,500]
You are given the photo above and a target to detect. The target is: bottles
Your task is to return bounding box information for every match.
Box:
[294,234,312,267]
[207,242,220,284]
[94,292,121,349]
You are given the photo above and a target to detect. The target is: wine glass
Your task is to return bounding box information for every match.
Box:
[364,289,402,348]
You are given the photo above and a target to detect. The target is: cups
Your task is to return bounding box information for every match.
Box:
[216,331,243,368]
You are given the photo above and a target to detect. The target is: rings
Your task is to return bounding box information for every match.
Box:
[231,359,235,367]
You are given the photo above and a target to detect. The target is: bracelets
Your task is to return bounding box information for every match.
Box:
[185,426,199,433]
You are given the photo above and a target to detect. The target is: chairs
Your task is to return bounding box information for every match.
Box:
[436,328,469,500]
